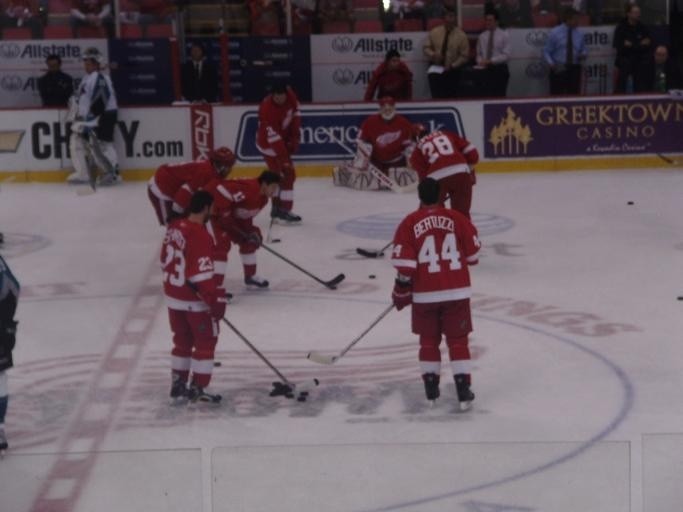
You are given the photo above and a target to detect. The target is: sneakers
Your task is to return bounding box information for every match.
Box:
[271,207,300,221]
[171,374,221,402]
[455,373,474,401]
[424,373,439,399]
[245,275,268,287]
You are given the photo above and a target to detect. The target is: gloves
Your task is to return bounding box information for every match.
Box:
[208,288,226,321]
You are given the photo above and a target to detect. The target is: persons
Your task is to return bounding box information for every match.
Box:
[212,166,279,287]
[365,50,413,102]
[68,47,118,185]
[410,129,479,223]
[277,0,428,36]
[37,56,76,108]
[423,8,470,96]
[255,79,302,219]
[392,181,482,402]
[148,146,236,227]
[475,14,512,96]
[545,6,669,95]
[69,2,114,40]
[161,189,225,406]
[1,0,48,43]
[180,41,214,94]
[357,96,426,178]
[0,230,21,456]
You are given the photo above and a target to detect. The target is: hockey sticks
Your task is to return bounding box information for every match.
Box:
[308,307,396,364]
[186,278,318,395]
[355,238,393,259]
[241,230,344,289]
[325,130,418,195]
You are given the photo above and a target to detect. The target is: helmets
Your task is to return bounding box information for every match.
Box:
[410,123,425,142]
[211,148,235,176]
